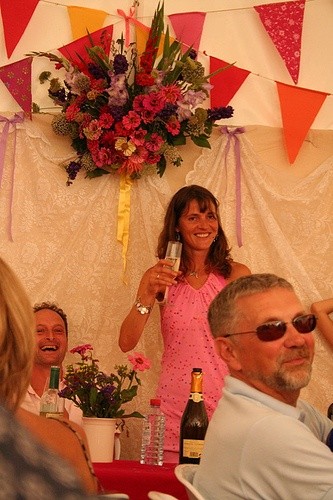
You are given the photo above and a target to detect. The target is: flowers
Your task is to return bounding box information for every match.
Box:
[57,340,152,419]
[23,0,237,186]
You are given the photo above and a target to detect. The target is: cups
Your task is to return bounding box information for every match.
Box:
[164,239,183,282]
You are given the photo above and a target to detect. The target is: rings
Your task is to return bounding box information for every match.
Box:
[157,272,159,278]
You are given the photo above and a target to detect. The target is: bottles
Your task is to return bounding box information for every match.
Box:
[42,366,60,414]
[179,369,209,465]
[140,399,165,465]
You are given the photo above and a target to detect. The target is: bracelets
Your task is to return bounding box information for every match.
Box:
[135,294,153,314]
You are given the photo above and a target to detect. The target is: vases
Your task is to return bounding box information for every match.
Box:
[81,416,117,463]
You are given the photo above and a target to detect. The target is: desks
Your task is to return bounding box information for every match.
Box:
[91,460,187,500]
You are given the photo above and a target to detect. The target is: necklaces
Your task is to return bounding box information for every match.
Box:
[189,265,207,279]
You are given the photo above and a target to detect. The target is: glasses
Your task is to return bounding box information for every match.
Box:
[223,313,319,342]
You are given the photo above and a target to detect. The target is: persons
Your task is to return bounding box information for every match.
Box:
[311,298,333,345]
[193,272,333,500]
[20,302,85,429]
[119,185,252,464]
[0,259,100,500]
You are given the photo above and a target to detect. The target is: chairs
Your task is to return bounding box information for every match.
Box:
[174,463,204,500]
